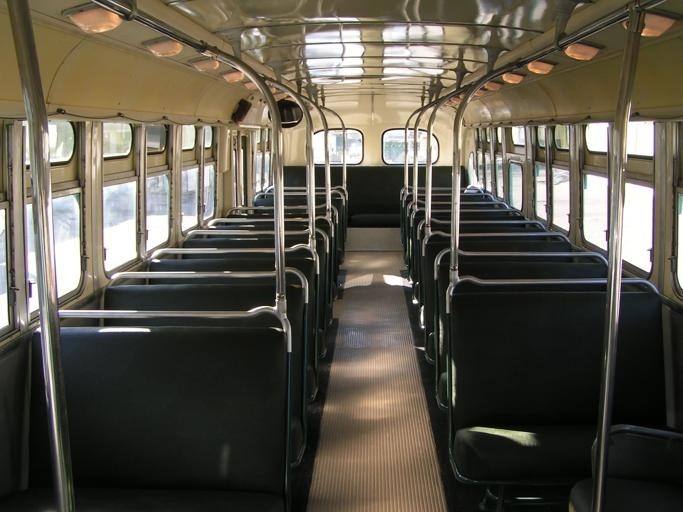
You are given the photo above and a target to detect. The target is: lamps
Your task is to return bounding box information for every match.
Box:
[440,9,675,112]
[60,1,289,101]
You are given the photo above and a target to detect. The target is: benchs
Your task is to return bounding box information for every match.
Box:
[398,184,683,512]
[30,185,349,512]
